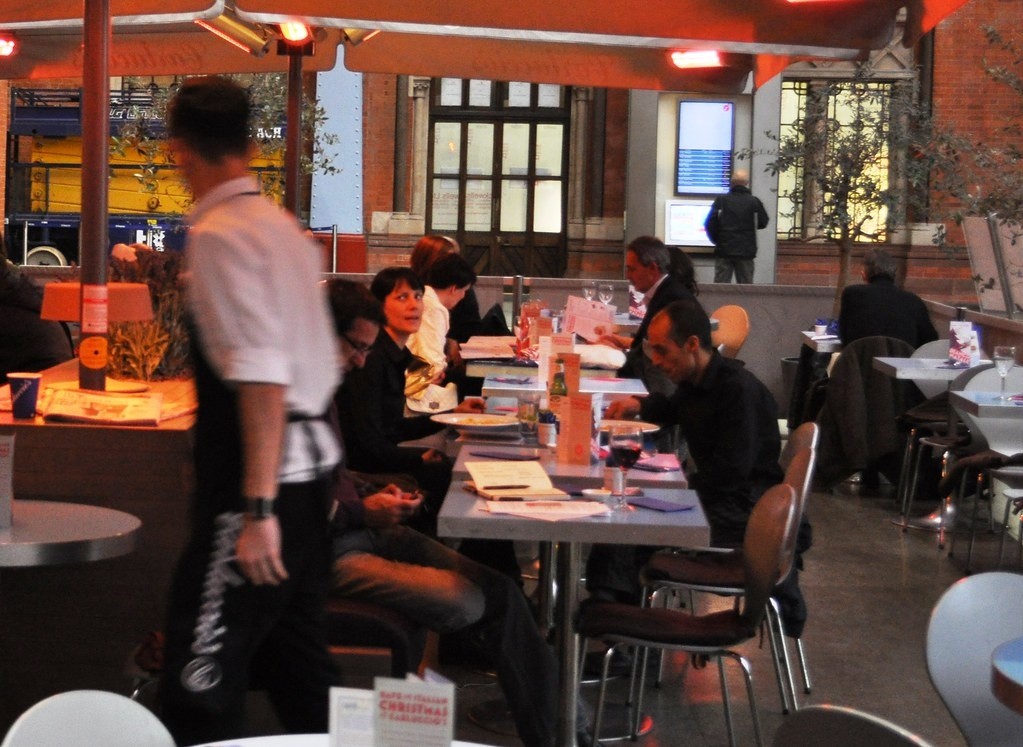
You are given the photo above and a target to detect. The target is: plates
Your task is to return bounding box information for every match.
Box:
[599,419,661,436]
[430,413,520,435]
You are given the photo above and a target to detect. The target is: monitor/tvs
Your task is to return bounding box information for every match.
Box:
[663,200,717,247]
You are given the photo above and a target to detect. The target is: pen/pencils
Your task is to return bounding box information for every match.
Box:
[499,495,570,501]
[483,485,530,490]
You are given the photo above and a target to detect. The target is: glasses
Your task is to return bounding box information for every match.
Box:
[340,332,371,353]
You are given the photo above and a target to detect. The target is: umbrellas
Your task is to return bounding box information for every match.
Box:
[1,0,970,402]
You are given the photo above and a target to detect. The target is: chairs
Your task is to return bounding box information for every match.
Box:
[574,421,817,747]
[827,337,919,503]
[904,362,1022,571]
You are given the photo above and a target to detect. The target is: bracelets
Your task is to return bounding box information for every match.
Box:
[243,496,274,521]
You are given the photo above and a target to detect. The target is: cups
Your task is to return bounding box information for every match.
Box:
[6,372,43,419]
[815,325,827,336]
[517,392,540,438]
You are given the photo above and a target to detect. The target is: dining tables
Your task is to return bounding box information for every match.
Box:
[950,389,1022,570]
[0,498,141,567]
[438,330,710,550]
[874,354,1008,535]
[804,328,846,386]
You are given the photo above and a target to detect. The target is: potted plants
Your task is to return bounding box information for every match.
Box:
[732,61,986,392]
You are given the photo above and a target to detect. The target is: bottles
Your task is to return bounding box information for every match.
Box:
[605,456,622,491]
[548,358,567,434]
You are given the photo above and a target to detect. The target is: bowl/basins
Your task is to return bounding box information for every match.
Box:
[581,489,612,502]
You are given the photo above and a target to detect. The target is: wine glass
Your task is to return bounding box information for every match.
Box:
[993,345,1017,401]
[583,280,597,301]
[609,424,643,512]
[599,284,614,305]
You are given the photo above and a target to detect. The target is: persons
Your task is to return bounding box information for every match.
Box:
[587,295,786,677]
[151,74,342,747]
[838,250,939,350]
[404,235,478,387]
[601,236,700,399]
[334,267,524,590]
[325,276,589,747]
[705,169,770,285]
